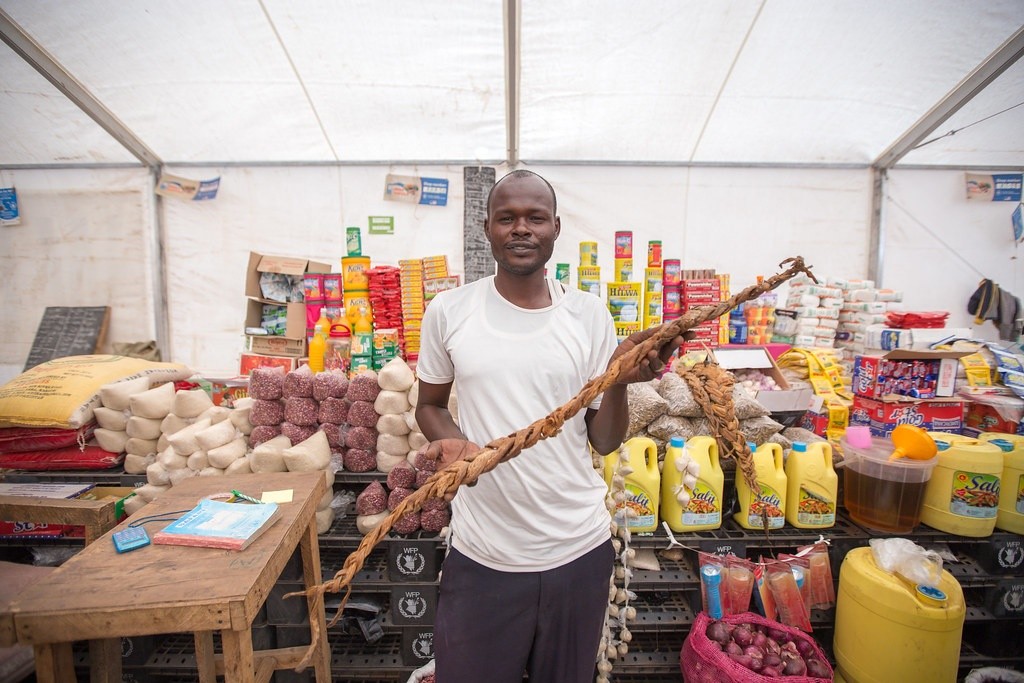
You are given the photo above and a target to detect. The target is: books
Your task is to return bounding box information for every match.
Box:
[151,498,283,552]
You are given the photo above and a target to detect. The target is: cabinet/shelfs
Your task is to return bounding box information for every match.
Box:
[12,467,331,683]
[72,466,1024,683]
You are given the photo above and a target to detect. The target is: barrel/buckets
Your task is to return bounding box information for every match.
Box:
[784,440,838,530]
[920,430,1004,538]
[599,434,662,537]
[660,434,726,534]
[976,432,1024,537]
[731,442,787,530]
[831,545,965,683]
[832,431,940,535]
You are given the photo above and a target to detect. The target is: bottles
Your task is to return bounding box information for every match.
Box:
[406,353,420,382]
[312,307,331,341]
[325,324,353,380]
[334,308,353,359]
[307,325,328,375]
[355,308,373,333]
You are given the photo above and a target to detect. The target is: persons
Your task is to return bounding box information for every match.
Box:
[416,168,693,683]
